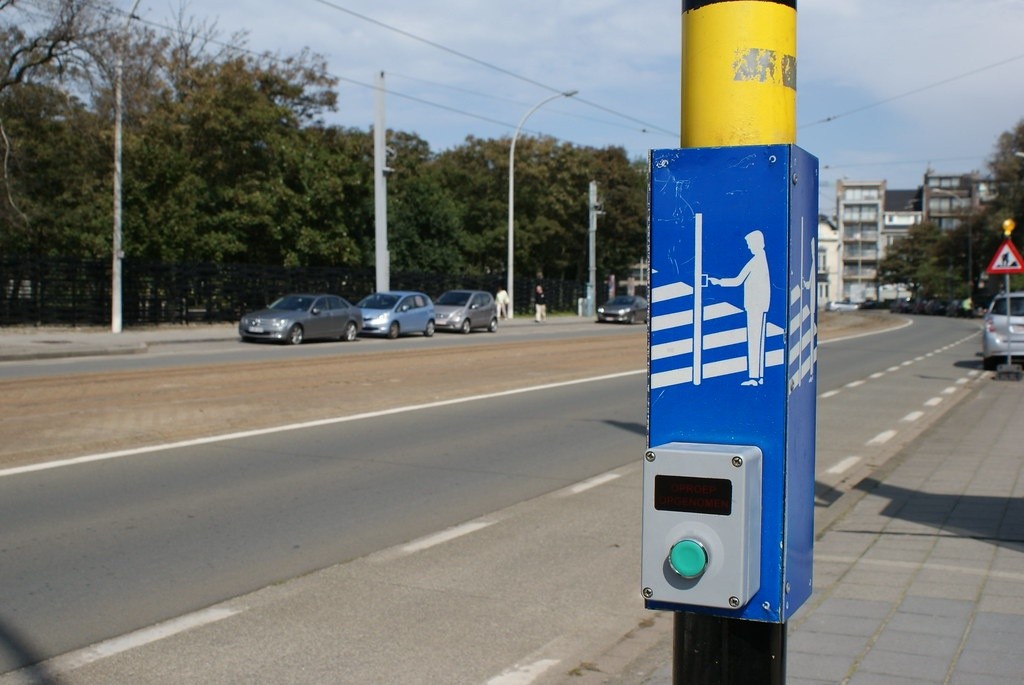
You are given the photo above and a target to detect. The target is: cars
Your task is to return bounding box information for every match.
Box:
[889,298,973,317]
[826,301,859,312]
[433,290,498,333]
[982,291,1024,371]
[355,291,436,339]
[596,295,648,325]
[238,293,364,345]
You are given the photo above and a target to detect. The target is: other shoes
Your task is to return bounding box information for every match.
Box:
[503,317,509,320]
[534,318,547,323]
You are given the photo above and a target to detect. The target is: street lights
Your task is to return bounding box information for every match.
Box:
[507,90,580,320]
[932,188,972,298]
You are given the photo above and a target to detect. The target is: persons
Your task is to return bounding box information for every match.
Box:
[533,284,547,322]
[494,285,509,324]
